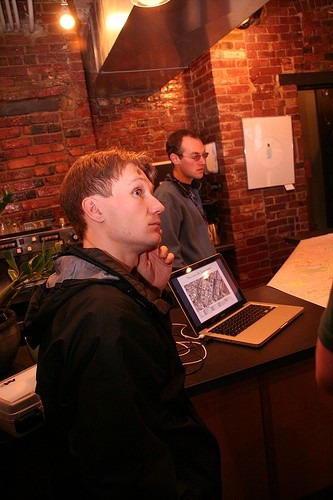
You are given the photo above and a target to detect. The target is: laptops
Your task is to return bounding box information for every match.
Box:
[168,253,304,347]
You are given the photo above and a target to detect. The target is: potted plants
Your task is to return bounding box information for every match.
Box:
[0,185,63,366]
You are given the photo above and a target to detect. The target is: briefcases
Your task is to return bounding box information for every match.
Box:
[0,364,44,438]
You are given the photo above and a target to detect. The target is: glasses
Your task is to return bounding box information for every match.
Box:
[175,151,208,161]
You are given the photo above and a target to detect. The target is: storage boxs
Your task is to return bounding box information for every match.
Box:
[0,363,44,438]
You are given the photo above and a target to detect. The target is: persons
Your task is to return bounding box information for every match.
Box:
[24,150,221,500]
[150,128,216,282]
[315,282,333,384]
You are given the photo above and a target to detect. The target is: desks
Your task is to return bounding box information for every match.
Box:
[0,284,326,500]
